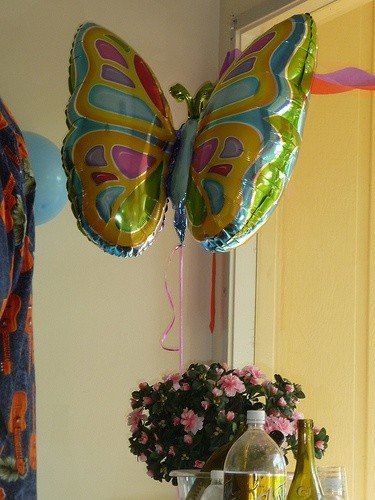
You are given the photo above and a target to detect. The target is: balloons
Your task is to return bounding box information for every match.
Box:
[61,12,317,258]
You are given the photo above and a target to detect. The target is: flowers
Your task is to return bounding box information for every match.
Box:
[127,362,329,482]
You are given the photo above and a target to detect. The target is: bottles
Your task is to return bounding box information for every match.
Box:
[223,410,287,500]
[286,418,324,500]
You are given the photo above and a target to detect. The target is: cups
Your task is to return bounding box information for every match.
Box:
[317,466,348,500]
[169,469,223,500]
